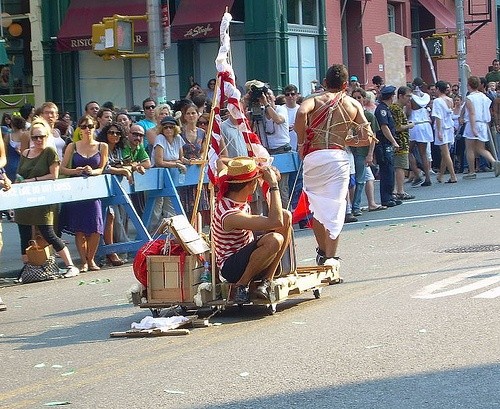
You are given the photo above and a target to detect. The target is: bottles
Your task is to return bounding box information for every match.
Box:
[200,262,211,283]
[16,174,24,181]
[179,164,185,184]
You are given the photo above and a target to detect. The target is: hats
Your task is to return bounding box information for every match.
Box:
[380,85,397,96]
[350,76,357,80]
[160,116,180,126]
[0,126,11,133]
[219,156,274,184]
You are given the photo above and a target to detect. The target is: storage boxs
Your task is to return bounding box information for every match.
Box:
[146,255,214,302]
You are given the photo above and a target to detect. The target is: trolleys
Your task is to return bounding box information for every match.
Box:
[128,158,329,315]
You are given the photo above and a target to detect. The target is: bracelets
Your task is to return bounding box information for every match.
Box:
[35,177,37,181]
[268,187,279,192]
[265,104,271,109]
[176,162,180,168]
[297,140,305,146]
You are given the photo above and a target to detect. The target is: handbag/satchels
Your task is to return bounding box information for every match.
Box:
[14,256,63,284]
[25,235,50,265]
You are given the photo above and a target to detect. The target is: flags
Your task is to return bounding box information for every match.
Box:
[205,12,273,186]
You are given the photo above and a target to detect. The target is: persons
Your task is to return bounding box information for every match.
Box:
[388,85,416,200]
[485,59,500,82]
[343,76,500,180]
[344,174,360,223]
[275,77,327,229]
[431,80,458,184]
[463,76,500,179]
[180,74,291,239]
[0,96,185,311]
[364,162,388,212]
[351,87,380,216]
[404,77,434,187]
[294,65,373,279]
[212,156,292,305]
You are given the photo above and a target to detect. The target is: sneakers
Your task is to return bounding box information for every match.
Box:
[255,278,271,300]
[234,284,251,304]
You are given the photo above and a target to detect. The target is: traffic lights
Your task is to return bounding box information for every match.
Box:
[104,17,134,53]
[423,37,445,58]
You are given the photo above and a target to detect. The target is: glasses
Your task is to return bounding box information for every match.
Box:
[145,105,156,110]
[351,80,356,82]
[79,124,95,130]
[164,125,175,129]
[107,130,121,136]
[31,135,46,141]
[452,89,458,91]
[353,96,362,99]
[130,131,144,138]
[285,93,295,97]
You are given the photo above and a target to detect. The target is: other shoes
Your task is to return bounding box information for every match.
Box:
[344,192,416,224]
[61,255,129,278]
[404,160,500,186]
[316,248,341,283]
